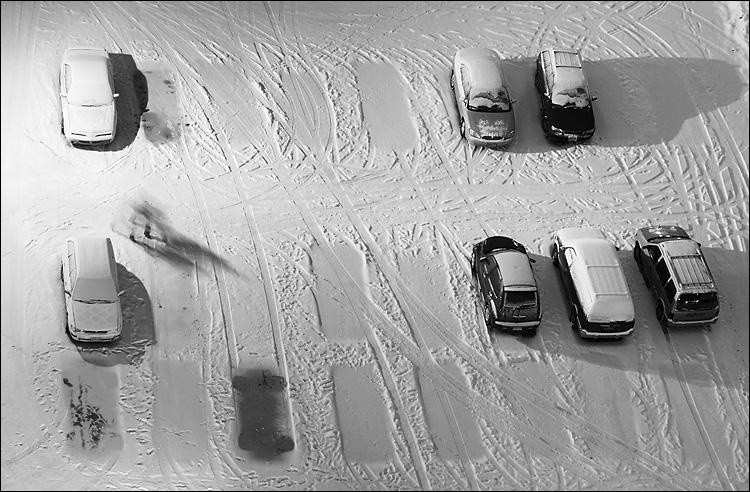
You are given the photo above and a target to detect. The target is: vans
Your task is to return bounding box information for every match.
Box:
[450,47,516,149]
[535,50,596,147]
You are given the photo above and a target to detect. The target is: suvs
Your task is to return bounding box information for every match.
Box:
[550,227,635,343]
[632,225,719,330]
[470,235,541,335]
[59,234,127,346]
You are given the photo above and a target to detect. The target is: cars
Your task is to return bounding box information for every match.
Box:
[60,44,118,150]
